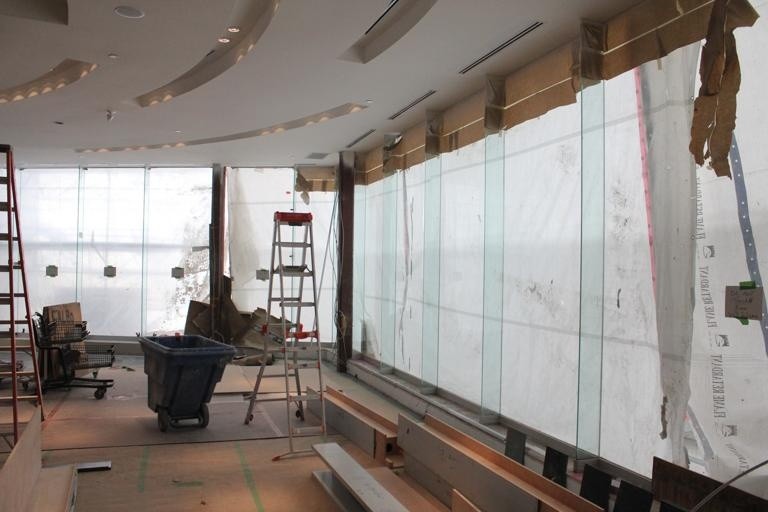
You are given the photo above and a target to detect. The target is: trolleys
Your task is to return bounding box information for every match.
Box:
[26,312,117,402]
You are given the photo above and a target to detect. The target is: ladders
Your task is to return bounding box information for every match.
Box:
[245,211,328,462]
[0,143,44,456]
